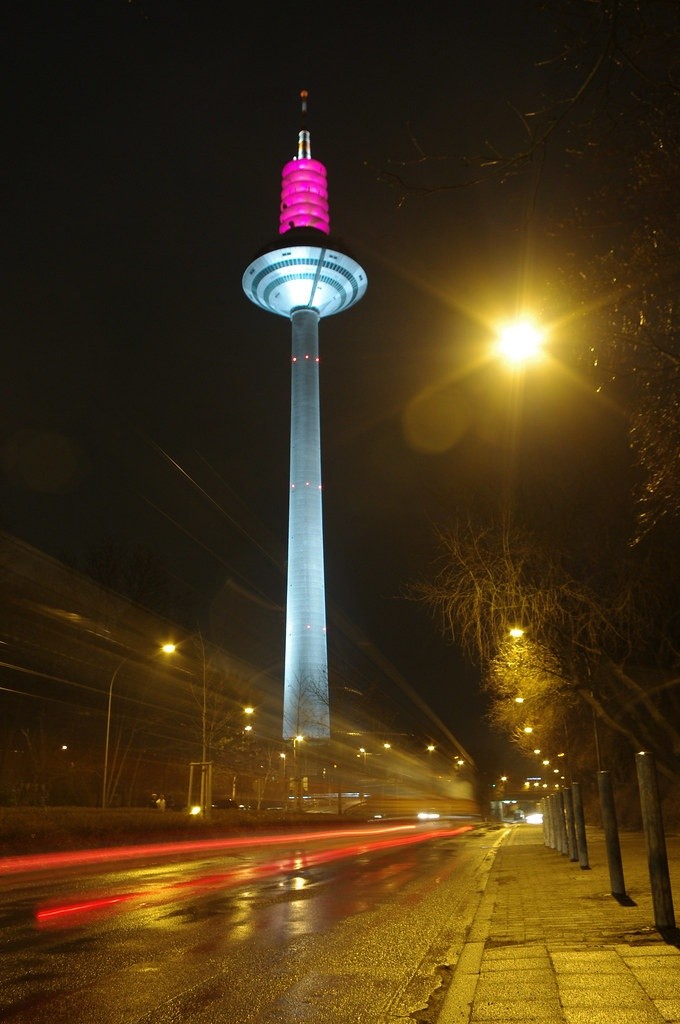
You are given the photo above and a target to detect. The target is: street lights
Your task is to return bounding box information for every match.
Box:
[204,707,255,811]
[515,692,589,868]
[101,644,177,809]
[509,626,627,895]
